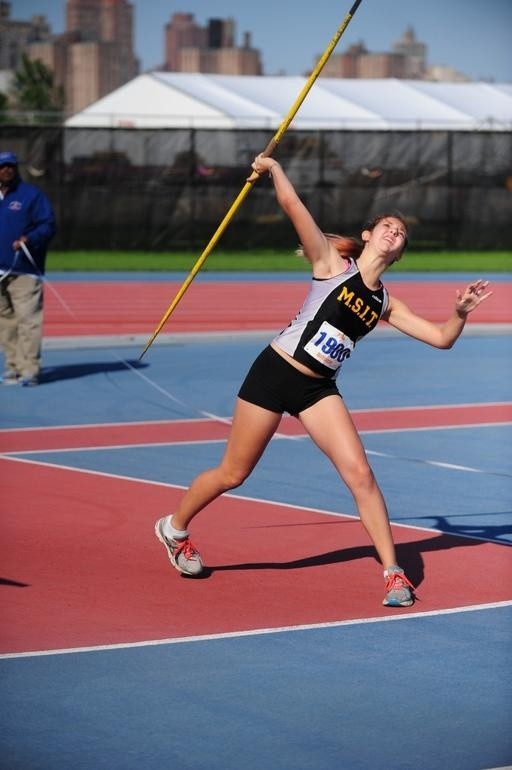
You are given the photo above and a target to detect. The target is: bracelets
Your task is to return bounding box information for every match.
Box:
[268,161,278,179]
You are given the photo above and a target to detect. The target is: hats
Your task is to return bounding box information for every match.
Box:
[0,151,19,167]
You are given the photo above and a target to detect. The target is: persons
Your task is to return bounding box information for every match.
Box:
[0,149,57,393]
[152,153,494,612]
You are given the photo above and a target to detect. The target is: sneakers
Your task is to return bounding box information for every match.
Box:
[0,370,41,388]
[154,514,206,577]
[381,562,416,608]
